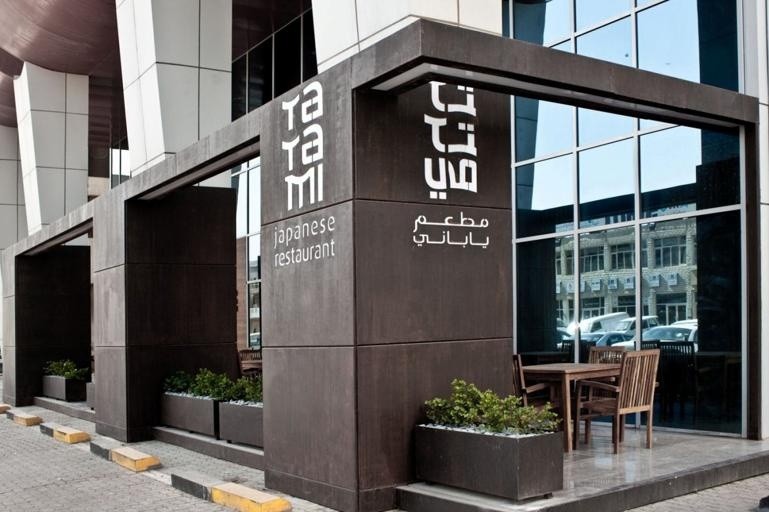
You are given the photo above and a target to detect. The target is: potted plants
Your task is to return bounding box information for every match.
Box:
[162,367,263,448]
[415,379,563,502]
[42,360,89,402]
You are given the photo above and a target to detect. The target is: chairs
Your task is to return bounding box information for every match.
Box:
[512,340,740,455]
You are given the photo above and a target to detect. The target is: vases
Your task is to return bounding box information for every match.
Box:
[86,382,95,410]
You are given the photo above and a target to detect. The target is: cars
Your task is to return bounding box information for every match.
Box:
[556,312,697,353]
